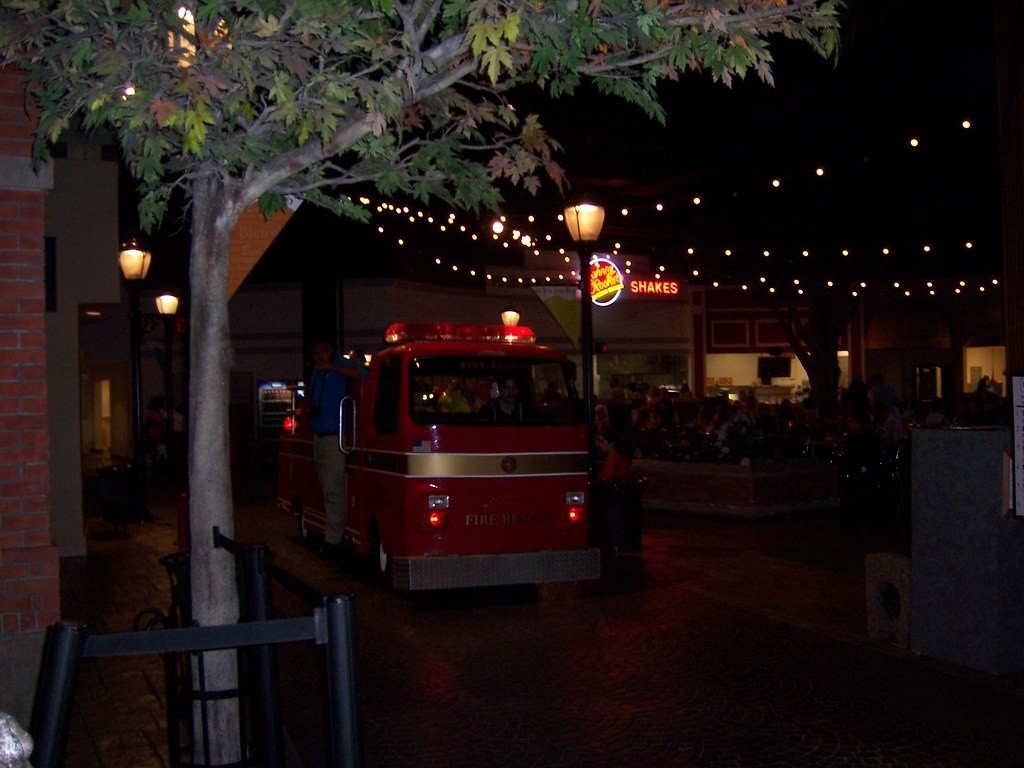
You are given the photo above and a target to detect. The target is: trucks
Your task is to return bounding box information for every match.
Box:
[272,310,602,592]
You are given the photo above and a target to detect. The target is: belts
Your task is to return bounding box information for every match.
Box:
[317,432,336,437]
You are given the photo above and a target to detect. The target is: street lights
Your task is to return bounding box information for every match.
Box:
[561,201,628,598]
[116,237,155,525]
[153,289,181,443]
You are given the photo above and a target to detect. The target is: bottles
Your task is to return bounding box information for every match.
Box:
[263,388,292,424]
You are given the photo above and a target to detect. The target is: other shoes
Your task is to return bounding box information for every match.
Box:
[317,546,343,555]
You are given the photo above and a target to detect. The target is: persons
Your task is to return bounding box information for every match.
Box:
[477,376,538,421]
[311,345,351,559]
[592,373,1007,467]
[592,427,642,550]
[441,369,488,413]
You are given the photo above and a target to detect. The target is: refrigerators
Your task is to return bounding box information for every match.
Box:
[256,381,304,466]
